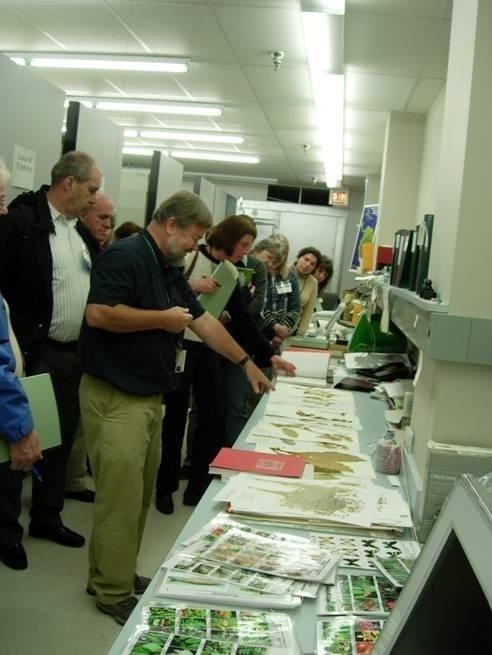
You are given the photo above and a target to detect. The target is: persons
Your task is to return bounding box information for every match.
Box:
[0,149,105,569]
[264,232,302,344]
[152,214,299,516]
[77,189,276,626]
[0,160,45,518]
[73,185,114,273]
[291,246,323,337]
[225,211,268,448]
[314,252,336,295]
[99,214,116,246]
[246,237,287,314]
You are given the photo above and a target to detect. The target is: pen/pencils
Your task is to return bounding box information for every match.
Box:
[32,465,44,483]
[201,274,222,288]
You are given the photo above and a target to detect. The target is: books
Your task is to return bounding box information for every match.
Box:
[207,446,307,482]
[219,462,313,485]
[275,344,333,389]
[289,335,330,350]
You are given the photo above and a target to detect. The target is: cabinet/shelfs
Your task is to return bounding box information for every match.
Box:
[235,197,348,297]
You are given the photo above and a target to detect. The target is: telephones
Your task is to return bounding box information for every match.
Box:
[356,362,405,382]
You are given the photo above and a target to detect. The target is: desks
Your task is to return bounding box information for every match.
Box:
[102,333,421,655]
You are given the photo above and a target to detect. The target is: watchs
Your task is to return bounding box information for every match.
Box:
[235,353,254,372]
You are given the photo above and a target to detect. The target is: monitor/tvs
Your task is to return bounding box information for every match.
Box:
[370,472,492,655]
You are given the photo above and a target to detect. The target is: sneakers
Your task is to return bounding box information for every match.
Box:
[86,575,152,596]
[95,597,140,627]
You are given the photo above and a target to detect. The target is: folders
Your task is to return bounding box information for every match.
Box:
[390,212,435,293]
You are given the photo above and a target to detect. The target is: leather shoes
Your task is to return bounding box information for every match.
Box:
[28,523,86,548]
[156,489,176,516]
[0,536,28,571]
[63,488,98,505]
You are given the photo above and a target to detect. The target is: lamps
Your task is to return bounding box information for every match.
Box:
[0,49,190,75]
[123,127,246,146]
[122,142,260,166]
[65,93,227,117]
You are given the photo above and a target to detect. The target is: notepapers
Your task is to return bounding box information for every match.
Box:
[10,144,37,192]
